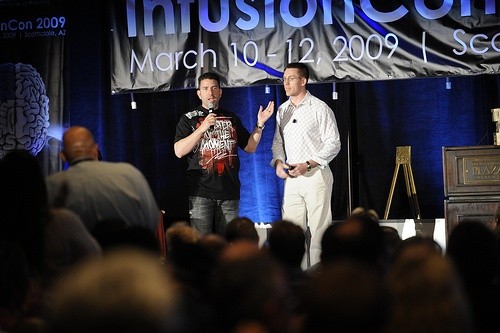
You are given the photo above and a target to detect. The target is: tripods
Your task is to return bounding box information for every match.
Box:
[383,146,422,219]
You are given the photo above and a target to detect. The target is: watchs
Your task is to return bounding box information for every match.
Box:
[256,124,265,130]
[306,161,312,171]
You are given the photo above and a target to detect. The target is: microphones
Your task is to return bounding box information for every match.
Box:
[208,101,215,132]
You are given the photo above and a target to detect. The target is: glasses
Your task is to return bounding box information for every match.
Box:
[282,76,302,82]
[352,207,379,222]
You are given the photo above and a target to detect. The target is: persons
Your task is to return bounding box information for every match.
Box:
[0,127,500,333]
[269,62,341,270]
[174,71,275,243]
